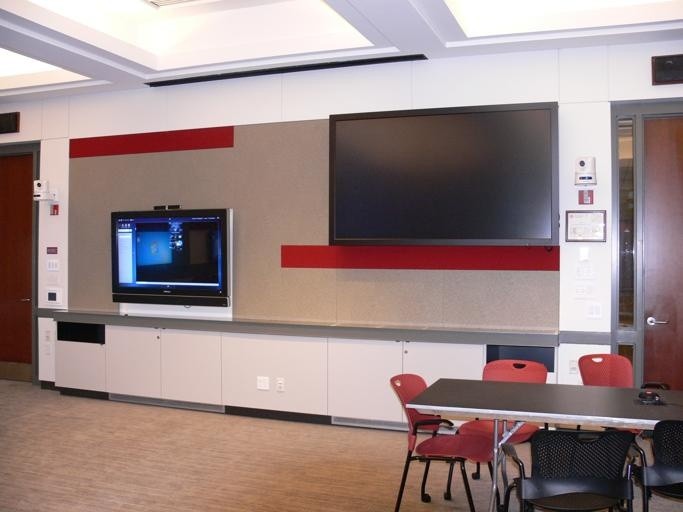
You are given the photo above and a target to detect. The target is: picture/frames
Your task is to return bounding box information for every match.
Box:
[565,209,608,243]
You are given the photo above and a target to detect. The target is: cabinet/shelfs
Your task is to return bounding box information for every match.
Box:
[220,319,331,427]
[105,313,224,415]
[329,325,487,439]
[49,310,108,400]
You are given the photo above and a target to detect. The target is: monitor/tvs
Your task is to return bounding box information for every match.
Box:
[329,101,559,247]
[111,208,230,307]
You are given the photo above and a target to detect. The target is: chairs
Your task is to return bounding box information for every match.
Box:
[390,372,502,512]
[628,419,683,512]
[637,381,683,443]
[496,427,640,512]
[442,358,551,508]
[574,354,651,502]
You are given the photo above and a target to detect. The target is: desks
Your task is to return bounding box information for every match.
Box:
[403,376,682,512]
